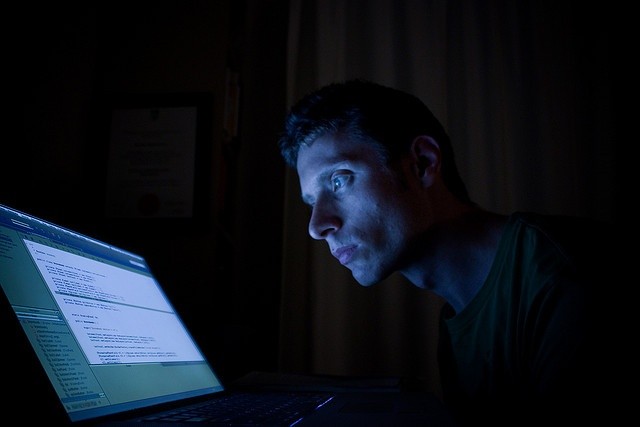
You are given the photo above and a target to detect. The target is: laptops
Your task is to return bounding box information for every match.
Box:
[0,203,354,425]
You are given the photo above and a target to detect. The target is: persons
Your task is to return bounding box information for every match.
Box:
[279,78,635,425]
